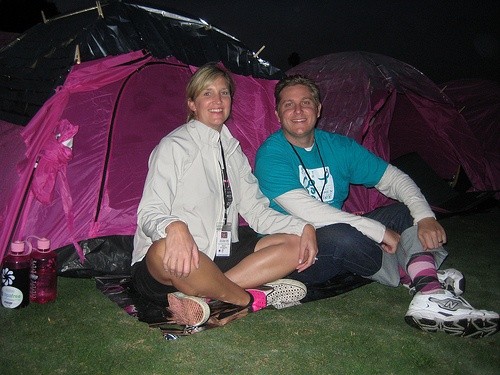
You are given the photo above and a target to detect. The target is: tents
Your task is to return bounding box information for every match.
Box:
[0,1,287,278]
[286,47,500,217]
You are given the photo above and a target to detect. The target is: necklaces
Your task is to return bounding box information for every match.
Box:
[287,138,327,203]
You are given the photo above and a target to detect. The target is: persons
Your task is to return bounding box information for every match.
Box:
[255,76,500,338]
[130,62,320,326]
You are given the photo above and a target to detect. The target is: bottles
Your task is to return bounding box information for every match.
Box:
[0,240,33,310]
[27,235,57,304]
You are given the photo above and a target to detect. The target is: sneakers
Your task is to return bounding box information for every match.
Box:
[167,292,212,326]
[244,277,306,312]
[405,289,500,338]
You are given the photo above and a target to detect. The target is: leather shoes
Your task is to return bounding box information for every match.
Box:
[403,267,464,297]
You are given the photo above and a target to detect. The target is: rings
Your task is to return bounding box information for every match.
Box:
[315,258,318,260]
[439,240,443,243]
